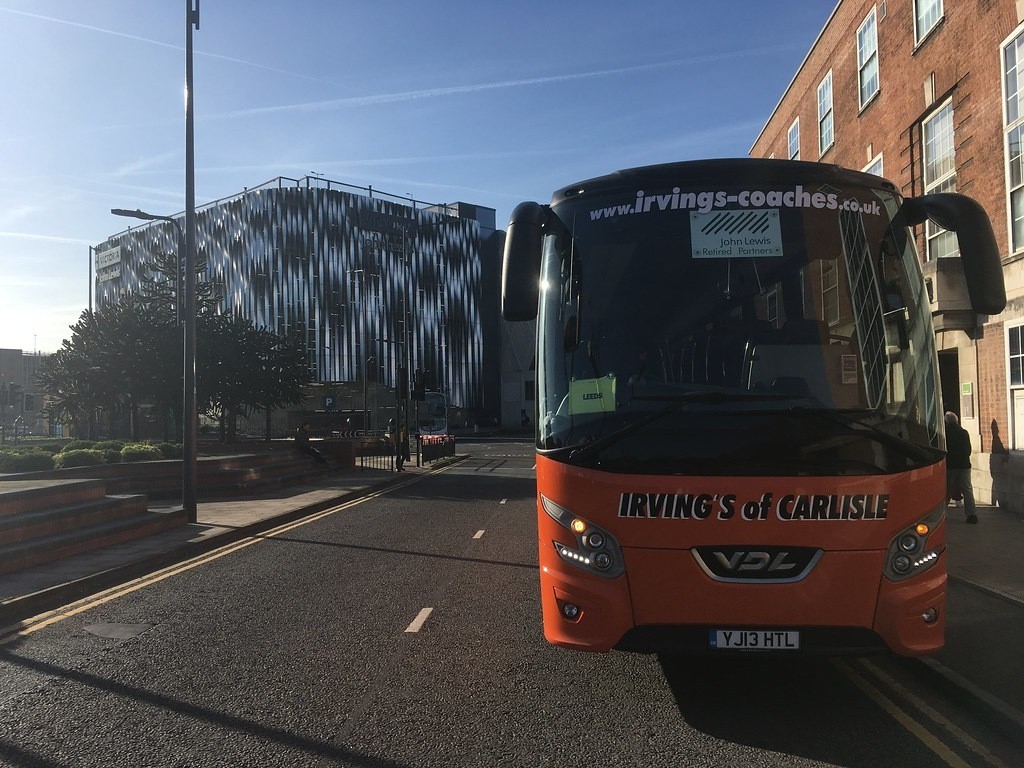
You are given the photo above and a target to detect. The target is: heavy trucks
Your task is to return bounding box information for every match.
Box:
[381,431,422,454]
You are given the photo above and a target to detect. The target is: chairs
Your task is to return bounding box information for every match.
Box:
[589,312,830,386]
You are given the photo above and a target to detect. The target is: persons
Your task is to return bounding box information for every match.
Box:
[945,410,978,523]
[296,423,328,463]
[395,423,408,471]
[521,413,530,430]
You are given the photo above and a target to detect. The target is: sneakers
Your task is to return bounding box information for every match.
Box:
[955,499,963,507]
[966,516,978,523]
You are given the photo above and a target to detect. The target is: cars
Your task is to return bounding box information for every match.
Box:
[347,430,383,448]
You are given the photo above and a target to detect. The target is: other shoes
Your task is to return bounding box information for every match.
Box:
[401,467,405,471]
[396,469,401,472]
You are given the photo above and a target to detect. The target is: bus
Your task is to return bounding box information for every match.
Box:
[500,158,1008,654]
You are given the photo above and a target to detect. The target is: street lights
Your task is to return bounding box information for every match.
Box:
[345,269,368,436]
[112,208,183,326]
